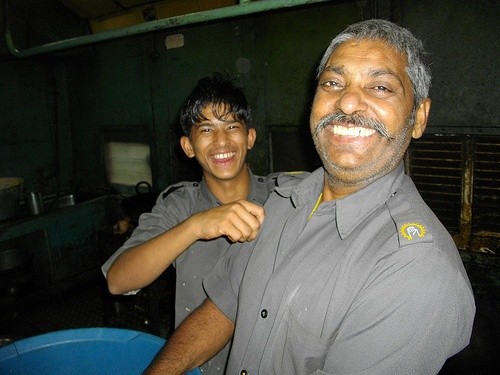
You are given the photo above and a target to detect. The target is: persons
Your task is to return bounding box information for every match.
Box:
[103,72,314,375]
[140,19,478,375]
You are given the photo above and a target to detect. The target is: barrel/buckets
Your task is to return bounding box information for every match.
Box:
[1,326,202,374]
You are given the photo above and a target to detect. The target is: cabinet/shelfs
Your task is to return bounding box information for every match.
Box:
[44,208,105,290]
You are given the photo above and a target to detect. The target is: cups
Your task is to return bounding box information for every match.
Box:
[27,190,45,216]
